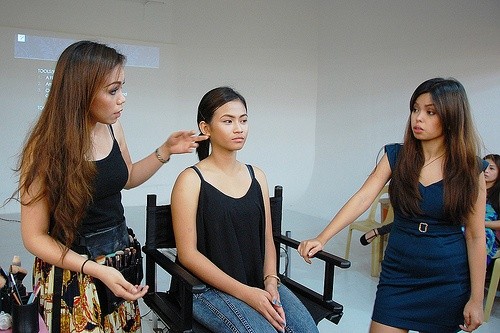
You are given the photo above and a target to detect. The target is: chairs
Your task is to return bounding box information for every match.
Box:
[345,184,395,277]
[144,185,351,333]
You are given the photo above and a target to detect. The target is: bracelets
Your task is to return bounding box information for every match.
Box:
[263,274,281,287]
[156,148,170,163]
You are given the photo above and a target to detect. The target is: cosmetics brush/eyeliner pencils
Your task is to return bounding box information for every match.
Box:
[9,256,41,304]
[94,247,136,270]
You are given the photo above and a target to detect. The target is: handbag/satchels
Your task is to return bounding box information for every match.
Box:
[93,238,144,317]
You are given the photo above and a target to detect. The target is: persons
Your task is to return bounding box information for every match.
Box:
[482,154,500,259]
[1,40,209,333]
[360,222,394,246]
[297,77,486,333]
[171,87,320,333]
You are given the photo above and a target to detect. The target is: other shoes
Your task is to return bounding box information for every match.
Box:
[360,228,377,246]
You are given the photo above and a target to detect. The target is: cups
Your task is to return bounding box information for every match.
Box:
[12,296,40,333]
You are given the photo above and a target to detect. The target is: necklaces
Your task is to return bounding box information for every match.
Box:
[423,152,445,168]
